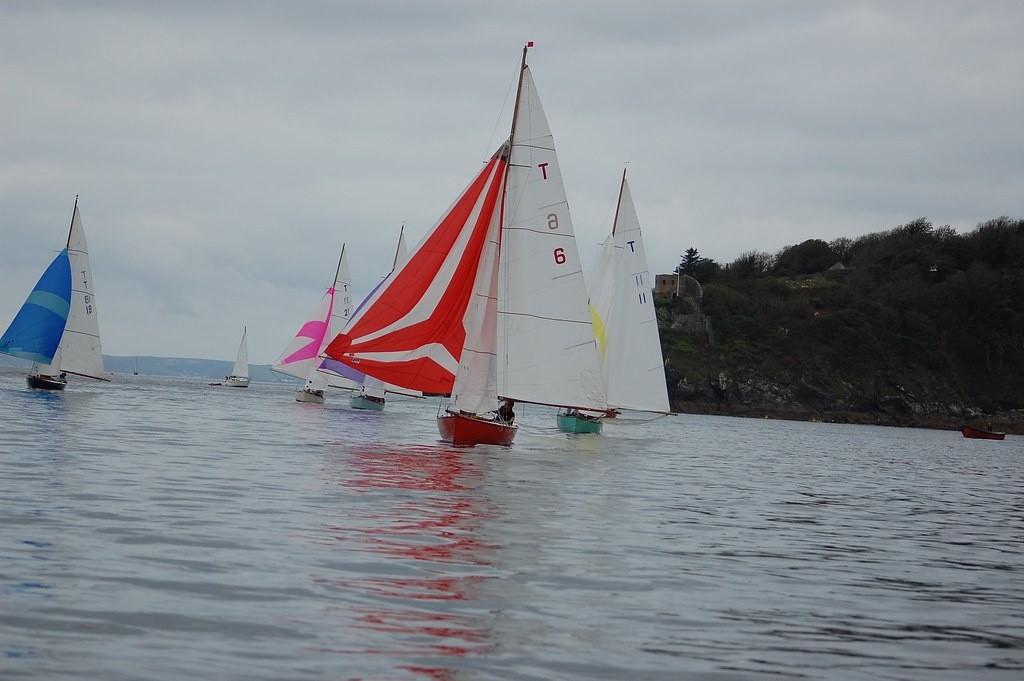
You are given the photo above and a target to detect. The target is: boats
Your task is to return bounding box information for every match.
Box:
[208,383,221,386]
[961,424,1005,440]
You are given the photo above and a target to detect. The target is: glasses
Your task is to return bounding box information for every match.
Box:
[505,403,511,406]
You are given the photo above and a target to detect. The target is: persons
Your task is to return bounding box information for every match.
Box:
[54,372,67,384]
[494,398,515,426]
[987,422,993,433]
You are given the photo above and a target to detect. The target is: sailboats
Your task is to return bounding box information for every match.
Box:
[0,195,112,390]
[225,326,250,387]
[316,225,427,411]
[556,161,678,434]
[269,242,360,403]
[319,42,622,445]
[133,355,138,375]
